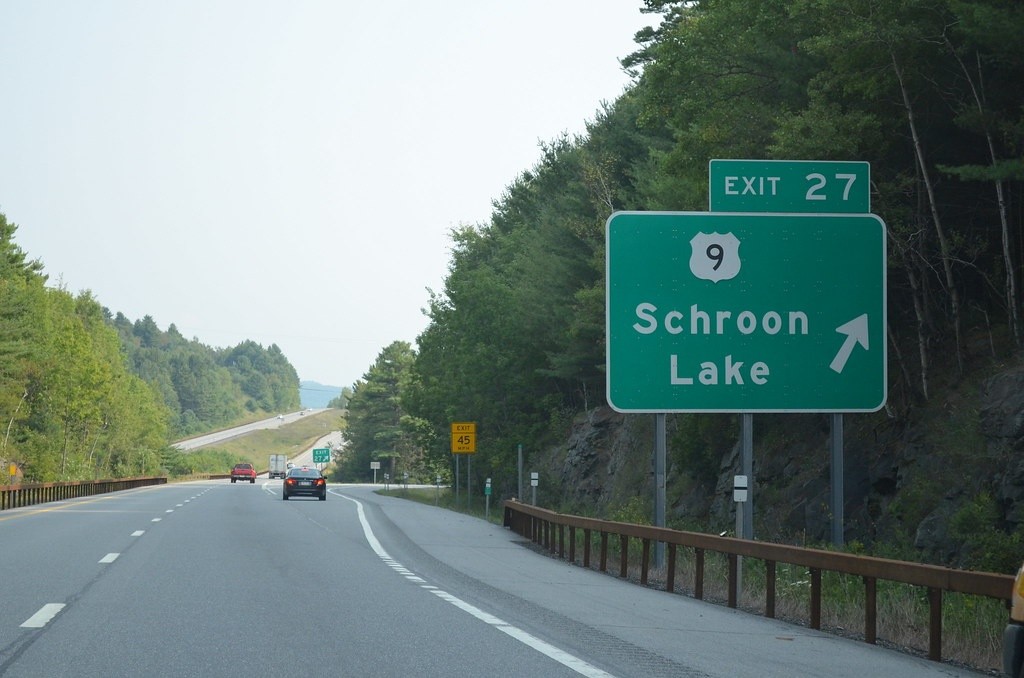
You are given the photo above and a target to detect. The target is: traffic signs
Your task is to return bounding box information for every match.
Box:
[605,210,887,413]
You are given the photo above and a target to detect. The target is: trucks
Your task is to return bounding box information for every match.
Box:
[269,454,288,479]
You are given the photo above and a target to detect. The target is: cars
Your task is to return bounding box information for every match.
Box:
[231,464,257,483]
[281,467,328,501]
[287,463,296,469]
